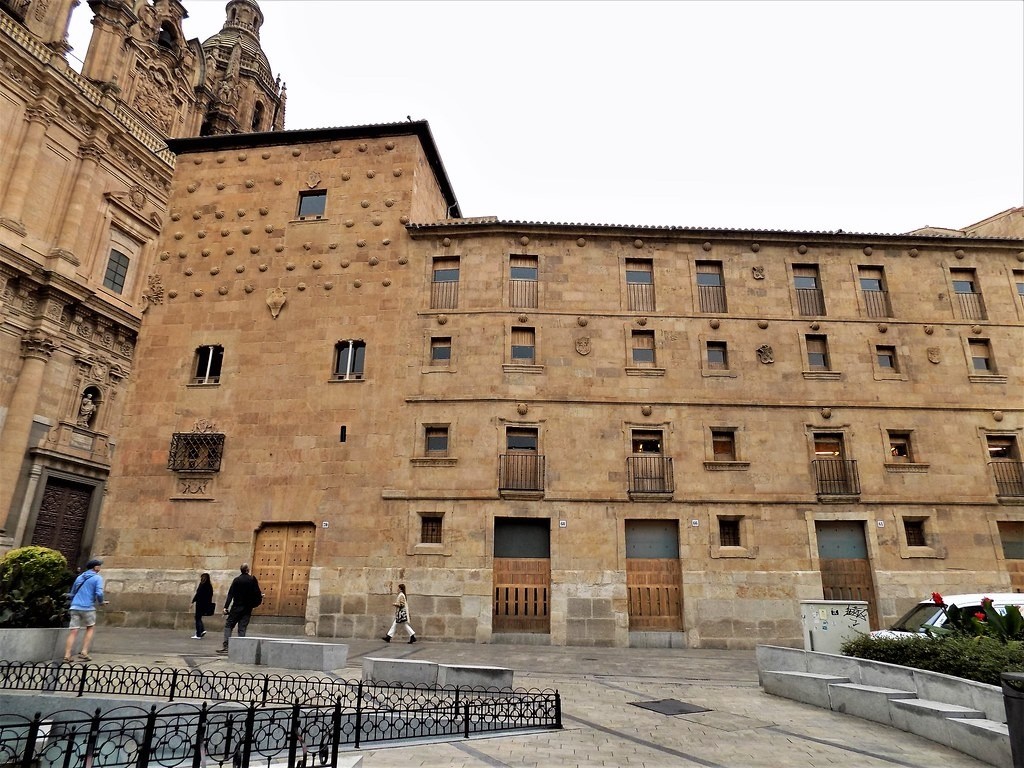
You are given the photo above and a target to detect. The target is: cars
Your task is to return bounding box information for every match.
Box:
[868,593,1024,653]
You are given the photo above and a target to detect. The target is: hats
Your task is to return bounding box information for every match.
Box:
[87,560,104,569]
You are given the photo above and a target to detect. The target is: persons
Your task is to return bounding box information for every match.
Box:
[382,584,417,644]
[62,559,110,662]
[76,394,96,427]
[216,563,262,653]
[190,573,213,639]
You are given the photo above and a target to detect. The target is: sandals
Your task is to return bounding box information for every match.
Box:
[78,653,92,660]
[62,657,74,662]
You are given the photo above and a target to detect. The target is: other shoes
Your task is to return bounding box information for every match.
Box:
[381,635,391,643]
[216,648,228,654]
[201,630,207,637]
[191,635,201,639]
[408,634,417,644]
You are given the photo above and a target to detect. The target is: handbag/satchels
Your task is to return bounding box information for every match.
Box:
[395,605,407,623]
[202,603,215,616]
[253,576,262,609]
